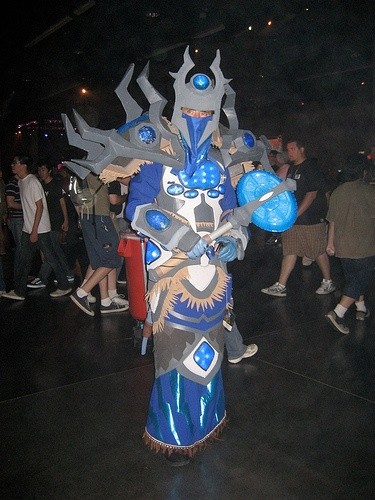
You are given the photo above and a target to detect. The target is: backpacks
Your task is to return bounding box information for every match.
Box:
[70,174,104,205]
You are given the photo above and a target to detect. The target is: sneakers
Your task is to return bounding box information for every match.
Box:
[315,281,337,295]
[100,300,130,314]
[69,291,95,317]
[355,308,371,320]
[2,290,26,301]
[324,310,350,334]
[228,343,259,364]
[26,277,47,288]
[260,283,288,297]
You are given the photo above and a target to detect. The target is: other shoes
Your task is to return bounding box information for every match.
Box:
[53,273,75,283]
[76,287,96,304]
[49,287,72,297]
[110,295,130,306]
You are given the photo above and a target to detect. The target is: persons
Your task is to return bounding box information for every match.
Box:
[0,132,375,363]
[125,95,249,466]
